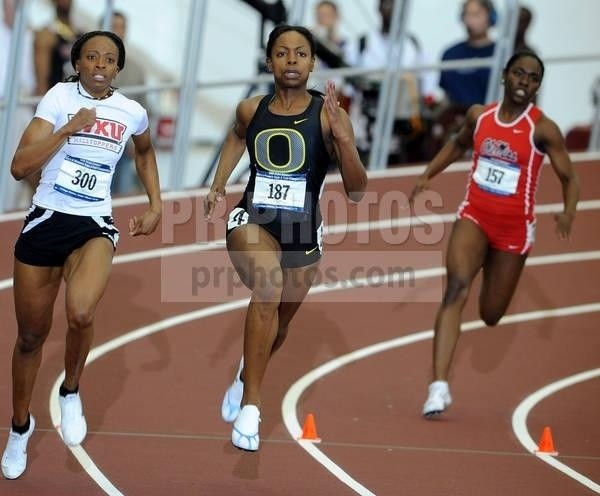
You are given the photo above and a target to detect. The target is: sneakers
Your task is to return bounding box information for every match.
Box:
[423,381,451,417]
[57,381,86,447]
[230,402,261,452]
[221,355,243,422]
[0,411,35,481]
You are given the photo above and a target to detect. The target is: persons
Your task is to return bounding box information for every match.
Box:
[0,0,150,195]
[306,0,532,166]
[407,51,581,417]
[0,30,162,480]
[203,22,368,454]
